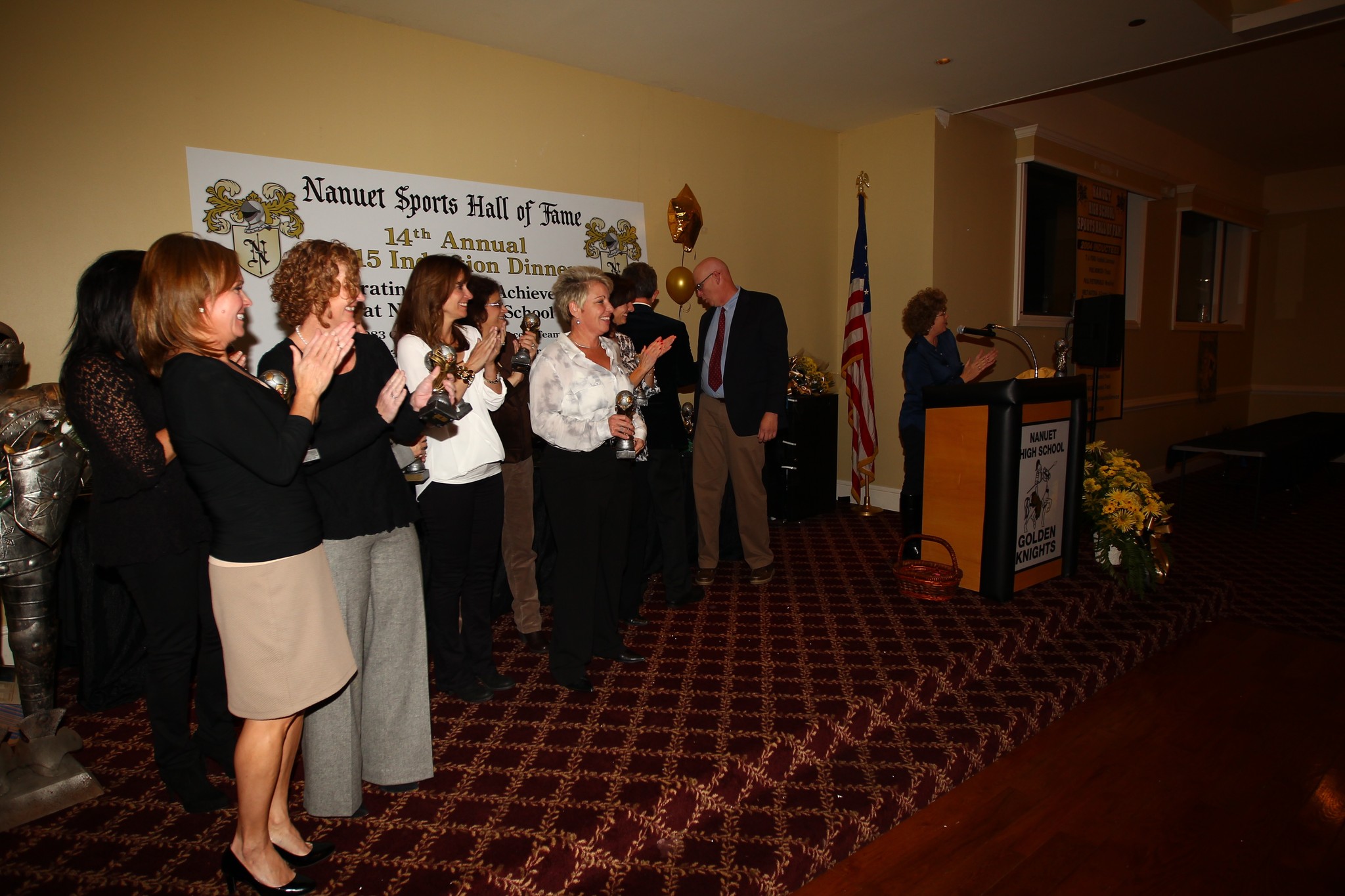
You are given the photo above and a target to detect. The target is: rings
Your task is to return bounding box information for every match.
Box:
[390,392,398,399]
[978,366,981,370]
[633,439,636,443]
[664,346,666,349]
[619,426,623,432]
[500,341,505,345]
[337,344,342,350]
[531,343,535,348]
[987,357,989,360]
[988,360,990,363]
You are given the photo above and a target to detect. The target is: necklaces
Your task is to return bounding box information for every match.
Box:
[295,324,308,345]
[569,333,600,349]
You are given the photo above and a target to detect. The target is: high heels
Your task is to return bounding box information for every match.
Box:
[221,844,318,896]
[273,837,336,867]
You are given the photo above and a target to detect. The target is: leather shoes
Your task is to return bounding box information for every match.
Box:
[626,616,651,626]
[611,649,646,664]
[665,584,706,607]
[552,669,592,692]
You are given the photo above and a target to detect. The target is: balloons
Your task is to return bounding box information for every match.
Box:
[666,266,695,308]
[668,183,704,253]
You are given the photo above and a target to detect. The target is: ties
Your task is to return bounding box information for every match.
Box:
[707,307,724,394]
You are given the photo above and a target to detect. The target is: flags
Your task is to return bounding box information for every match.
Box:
[839,191,879,506]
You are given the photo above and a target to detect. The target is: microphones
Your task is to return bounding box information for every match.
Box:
[957,323,997,338]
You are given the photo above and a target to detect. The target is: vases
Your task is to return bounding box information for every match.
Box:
[1092,532,1123,566]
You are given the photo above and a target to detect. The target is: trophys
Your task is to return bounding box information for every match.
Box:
[1053,337,1069,378]
[681,401,695,432]
[260,370,320,465]
[634,353,648,406]
[454,398,473,420]
[403,439,430,482]
[418,343,457,429]
[615,390,636,459]
[511,313,541,375]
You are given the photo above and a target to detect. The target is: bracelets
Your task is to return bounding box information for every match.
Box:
[455,365,476,388]
[646,365,655,375]
[482,368,501,384]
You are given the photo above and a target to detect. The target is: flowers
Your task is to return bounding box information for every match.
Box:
[1082,440,1174,601]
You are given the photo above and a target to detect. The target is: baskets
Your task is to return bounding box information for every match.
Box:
[890,534,965,603]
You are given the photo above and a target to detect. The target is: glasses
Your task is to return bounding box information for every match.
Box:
[695,272,721,291]
[484,300,504,309]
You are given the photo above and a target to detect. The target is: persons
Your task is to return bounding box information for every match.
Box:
[601,269,678,626]
[57,250,249,813]
[616,262,704,607]
[455,273,549,653]
[0,321,95,797]
[529,267,647,692]
[388,254,518,702]
[898,287,998,561]
[259,236,457,819]
[353,300,428,471]
[129,230,361,895]
[692,256,790,585]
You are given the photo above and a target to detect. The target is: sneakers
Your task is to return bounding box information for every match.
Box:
[482,672,517,692]
[694,565,718,585]
[519,629,550,654]
[747,562,776,584]
[457,681,494,703]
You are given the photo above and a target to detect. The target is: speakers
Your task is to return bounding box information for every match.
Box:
[1071,294,1125,367]
[763,395,838,520]
